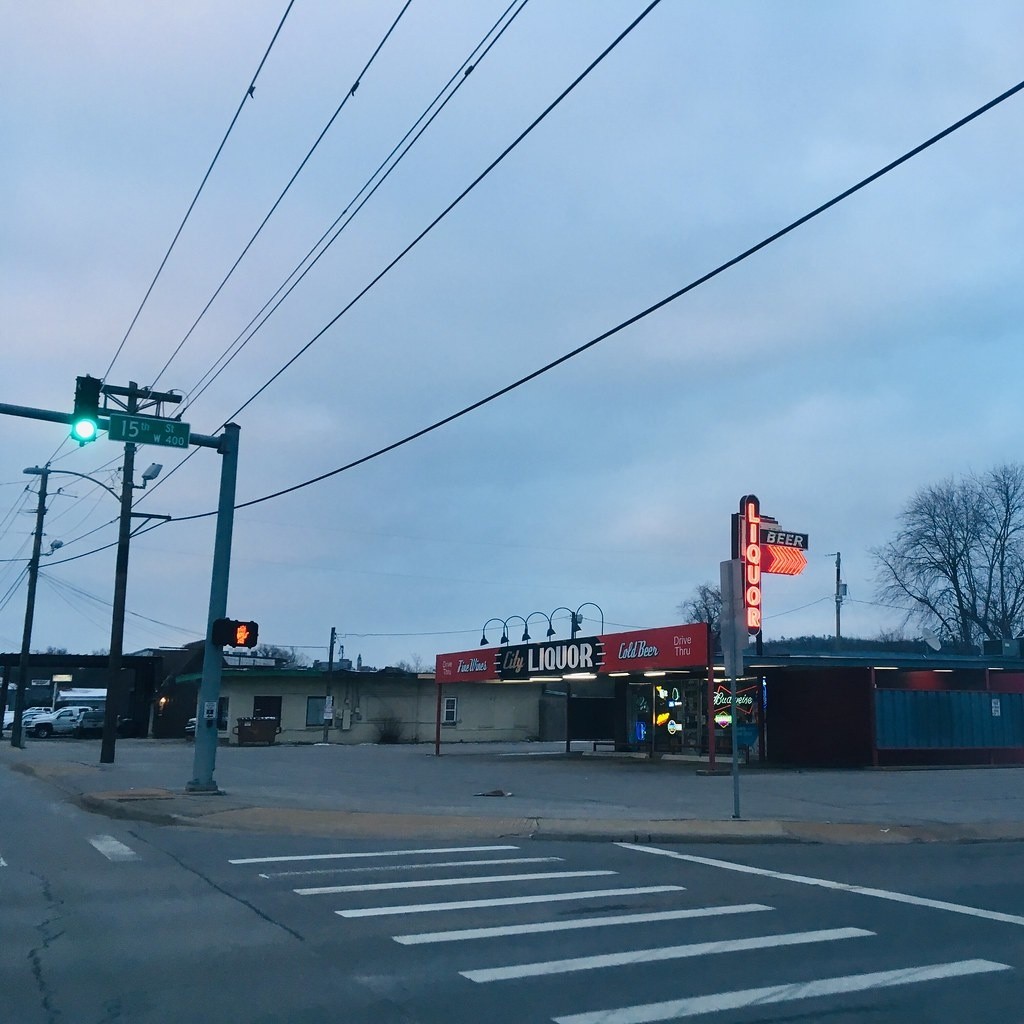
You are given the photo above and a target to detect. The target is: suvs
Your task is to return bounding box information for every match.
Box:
[31,705,92,739]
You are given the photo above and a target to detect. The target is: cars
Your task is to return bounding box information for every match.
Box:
[2,706,53,732]
[186,717,197,733]
[75,710,107,736]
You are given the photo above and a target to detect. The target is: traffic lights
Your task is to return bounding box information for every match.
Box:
[71,375,103,445]
[212,617,259,648]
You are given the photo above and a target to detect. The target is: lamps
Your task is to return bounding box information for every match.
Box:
[480,602,605,647]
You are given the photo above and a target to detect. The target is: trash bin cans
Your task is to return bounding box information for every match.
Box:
[232,717,282,747]
